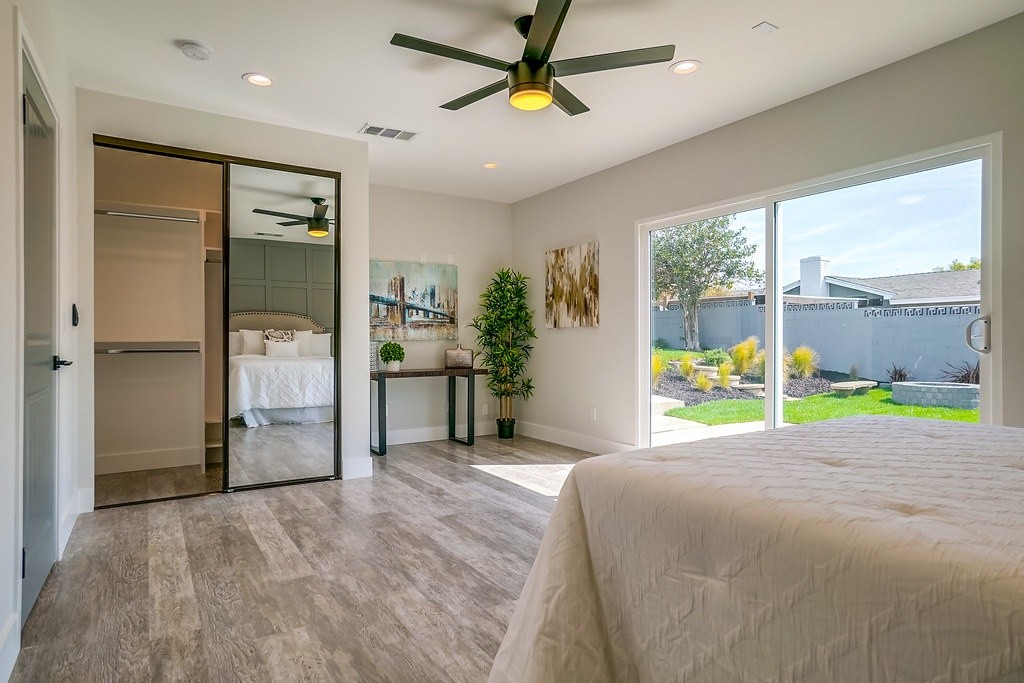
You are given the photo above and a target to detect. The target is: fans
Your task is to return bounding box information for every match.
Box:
[390,0,674,118]
[252,197,335,228]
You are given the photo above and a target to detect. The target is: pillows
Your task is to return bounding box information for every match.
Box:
[229,328,332,357]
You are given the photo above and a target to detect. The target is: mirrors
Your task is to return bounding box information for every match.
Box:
[218,161,340,490]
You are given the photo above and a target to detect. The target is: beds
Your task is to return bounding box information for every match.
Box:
[229,311,335,427]
[489,416,1024,683]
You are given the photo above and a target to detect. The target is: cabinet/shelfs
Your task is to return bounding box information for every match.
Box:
[94,202,226,475]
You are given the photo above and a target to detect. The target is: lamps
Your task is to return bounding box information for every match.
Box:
[507,61,554,110]
[307,218,329,237]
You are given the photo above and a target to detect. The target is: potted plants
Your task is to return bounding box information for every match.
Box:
[380,341,405,371]
[465,268,540,440]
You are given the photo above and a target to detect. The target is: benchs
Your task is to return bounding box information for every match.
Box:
[829,381,878,397]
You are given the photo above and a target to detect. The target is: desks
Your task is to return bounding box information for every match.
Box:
[371,367,488,457]
